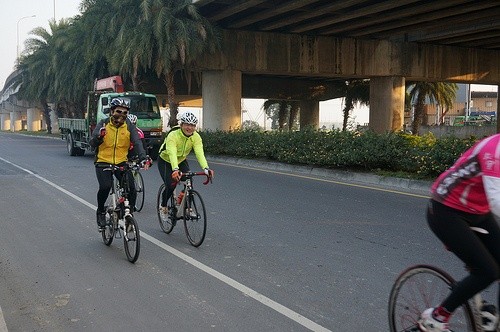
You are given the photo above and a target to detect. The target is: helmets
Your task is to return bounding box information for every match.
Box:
[181,113,198,126]
[127,114,137,123]
[111,97,131,109]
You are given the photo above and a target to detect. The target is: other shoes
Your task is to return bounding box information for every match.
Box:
[97,209,106,226]
[186,208,196,216]
[162,207,168,214]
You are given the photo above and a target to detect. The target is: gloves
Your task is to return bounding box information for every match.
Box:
[172,171,180,182]
[204,168,213,178]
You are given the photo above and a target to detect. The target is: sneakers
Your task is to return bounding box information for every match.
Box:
[419,308,452,332]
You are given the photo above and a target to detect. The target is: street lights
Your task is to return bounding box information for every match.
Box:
[17,15,36,69]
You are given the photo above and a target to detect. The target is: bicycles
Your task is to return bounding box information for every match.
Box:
[388,226,500,332]
[157,171,212,248]
[95,146,154,263]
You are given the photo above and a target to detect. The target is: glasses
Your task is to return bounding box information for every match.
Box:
[113,110,128,115]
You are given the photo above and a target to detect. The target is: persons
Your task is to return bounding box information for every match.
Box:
[88,98,150,227]
[419,133,500,332]
[157,114,213,221]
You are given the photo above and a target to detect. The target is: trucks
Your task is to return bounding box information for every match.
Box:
[61,76,163,155]
